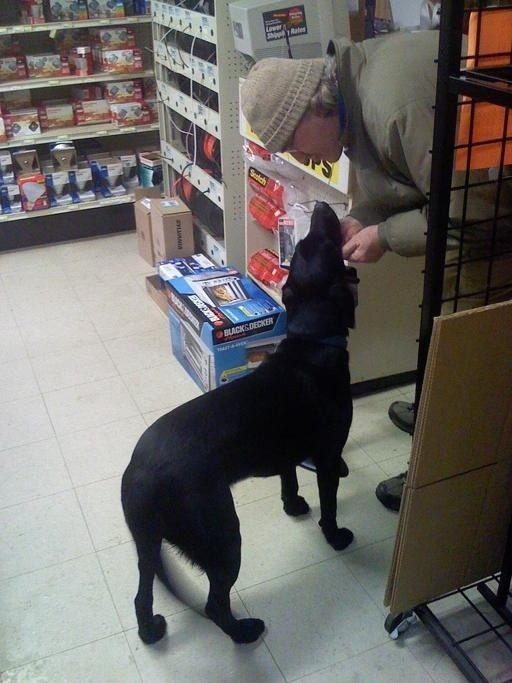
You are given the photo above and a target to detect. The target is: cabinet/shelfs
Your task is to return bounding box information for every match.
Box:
[380,1,512,683]
[0,0,157,251]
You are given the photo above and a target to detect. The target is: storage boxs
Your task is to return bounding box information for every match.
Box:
[150,196,194,266]
[143,253,289,392]
[134,187,161,263]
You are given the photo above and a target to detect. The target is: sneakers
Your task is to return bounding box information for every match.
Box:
[375,471,408,513]
[387,399,414,432]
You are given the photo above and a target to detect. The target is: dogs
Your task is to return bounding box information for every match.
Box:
[120,202,361,645]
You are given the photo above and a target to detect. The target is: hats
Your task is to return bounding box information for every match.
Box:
[236,56,325,154]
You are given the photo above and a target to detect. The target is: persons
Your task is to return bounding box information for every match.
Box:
[240,28,512,513]
